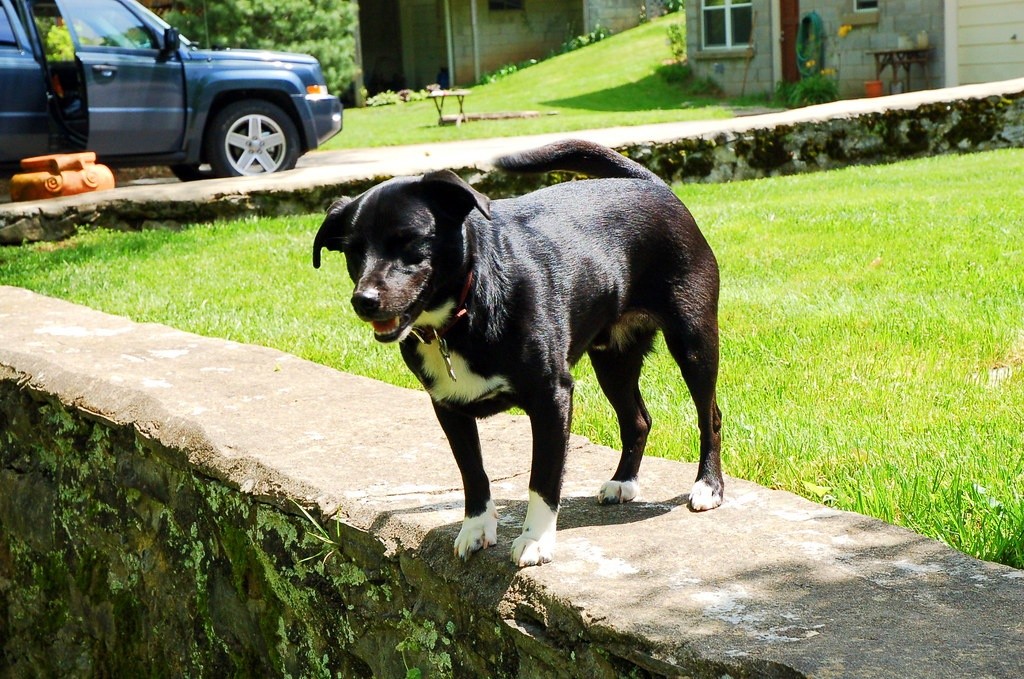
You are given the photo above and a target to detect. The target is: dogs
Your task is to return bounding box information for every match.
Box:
[312,138,727,570]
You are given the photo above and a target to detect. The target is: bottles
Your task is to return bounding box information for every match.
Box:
[916,30,928,49]
[897,33,911,50]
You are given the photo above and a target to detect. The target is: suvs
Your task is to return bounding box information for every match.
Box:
[0,0,345,182]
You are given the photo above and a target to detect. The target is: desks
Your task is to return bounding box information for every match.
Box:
[866,47,937,93]
[426,89,471,126]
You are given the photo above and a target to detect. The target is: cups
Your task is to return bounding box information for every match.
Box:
[907,41,914,50]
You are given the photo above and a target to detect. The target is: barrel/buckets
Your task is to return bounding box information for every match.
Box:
[864,80,882,98]
